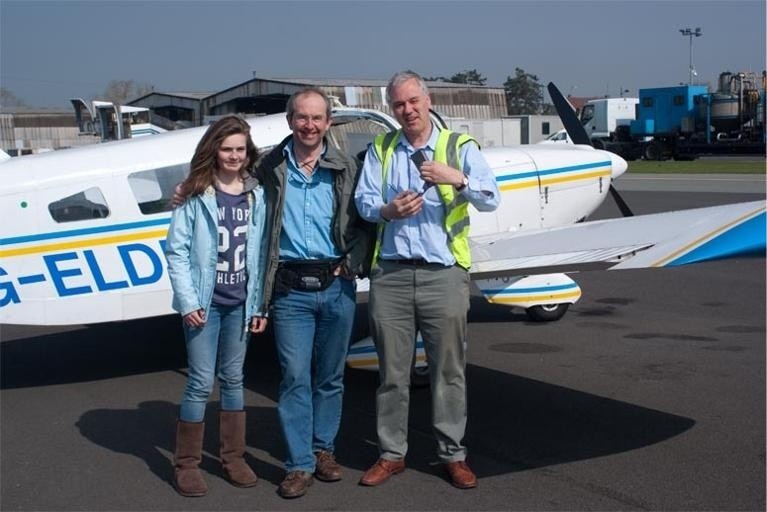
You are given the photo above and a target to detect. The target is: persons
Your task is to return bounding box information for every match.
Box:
[169,88,370,497]
[170,115,268,495]
[351,70,500,485]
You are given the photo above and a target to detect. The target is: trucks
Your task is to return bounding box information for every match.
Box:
[580,86,766,160]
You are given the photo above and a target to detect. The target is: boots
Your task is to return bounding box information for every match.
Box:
[171,419,209,498]
[217,410,257,488]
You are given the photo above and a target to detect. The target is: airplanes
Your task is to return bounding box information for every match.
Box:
[0,82,766,386]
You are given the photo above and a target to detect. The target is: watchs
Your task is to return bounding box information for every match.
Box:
[453,172,469,191]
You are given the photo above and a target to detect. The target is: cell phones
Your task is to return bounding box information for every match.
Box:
[411,148,430,174]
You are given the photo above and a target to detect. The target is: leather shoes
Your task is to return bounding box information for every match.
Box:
[441,461,478,491]
[359,457,406,486]
[277,448,344,499]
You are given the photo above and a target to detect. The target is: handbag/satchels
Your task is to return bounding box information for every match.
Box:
[275,254,340,293]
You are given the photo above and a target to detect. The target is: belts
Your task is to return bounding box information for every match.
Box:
[378,256,443,268]
